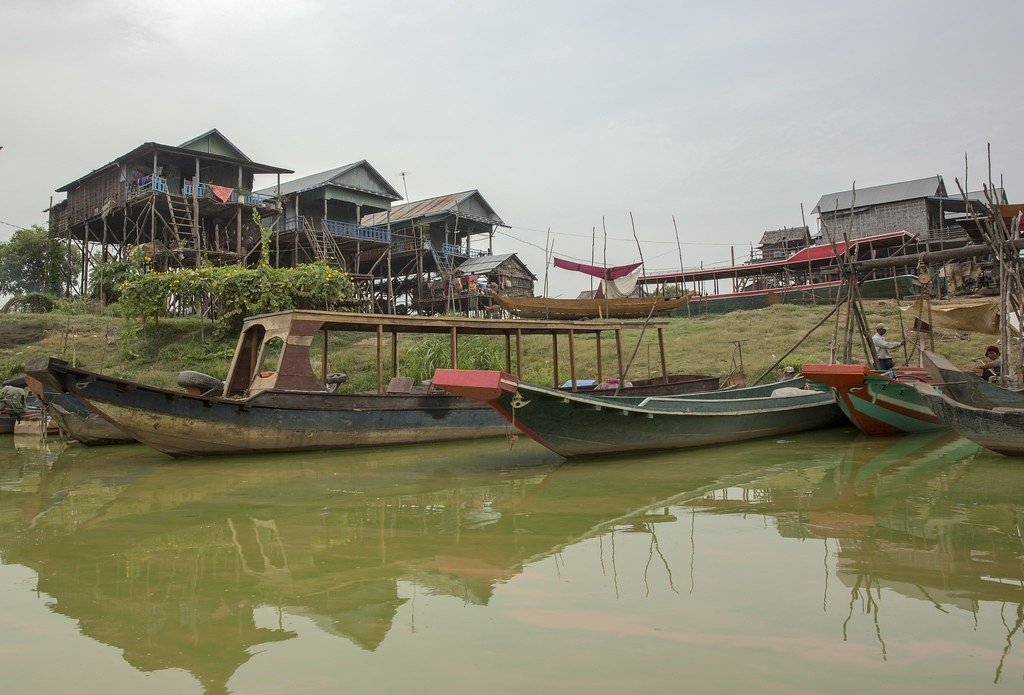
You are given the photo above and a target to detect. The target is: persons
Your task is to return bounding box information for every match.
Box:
[871,323,906,369]
[972,346,1002,383]
[452,271,498,294]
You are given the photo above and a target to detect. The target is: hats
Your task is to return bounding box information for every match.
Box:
[875,323,890,330]
[985,346,1001,357]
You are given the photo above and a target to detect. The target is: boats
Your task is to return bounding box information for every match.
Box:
[913,352,1023,460]
[425,367,852,462]
[25,376,142,447]
[802,362,988,437]
[25,309,720,456]
[488,286,697,319]
[628,232,932,317]
